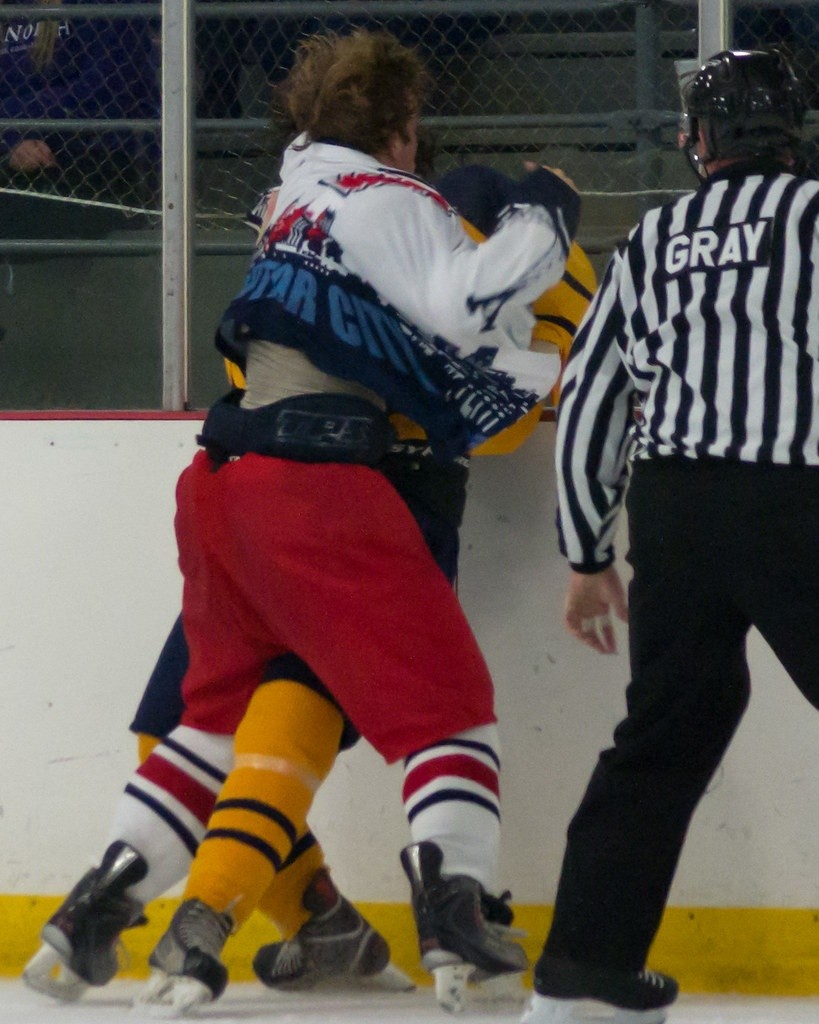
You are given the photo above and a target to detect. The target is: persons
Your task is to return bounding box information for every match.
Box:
[25,29,580,1015]
[130,169,598,1002]
[532,50,819,1021]
[1,139,59,173]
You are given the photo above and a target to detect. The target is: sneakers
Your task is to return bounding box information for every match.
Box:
[131,896,230,1014]
[528,945,681,1024]
[401,840,527,1014]
[22,841,150,1004]
[250,866,414,1000]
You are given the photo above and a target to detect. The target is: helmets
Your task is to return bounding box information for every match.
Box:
[682,44,804,174]
